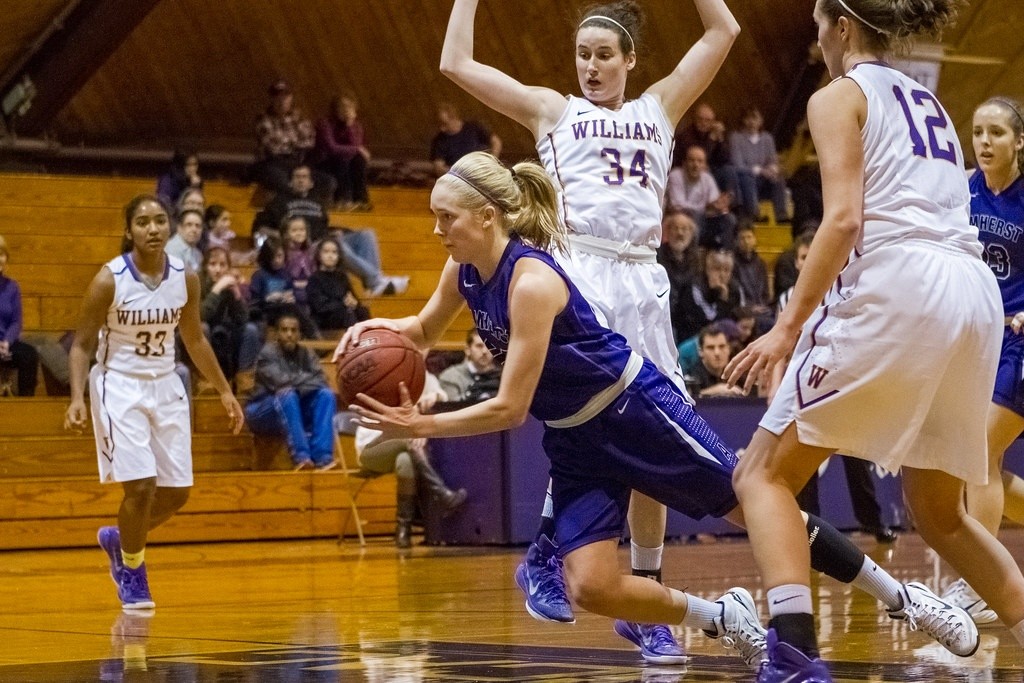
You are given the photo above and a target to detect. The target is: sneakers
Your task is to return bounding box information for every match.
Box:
[914,634,998,670]
[939,578,998,624]
[757,628,832,683]
[514,533,577,625]
[120,562,155,609]
[702,585,771,672]
[885,582,980,657]
[641,668,684,683]
[122,609,155,642]
[97,526,124,587]
[613,619,688,665]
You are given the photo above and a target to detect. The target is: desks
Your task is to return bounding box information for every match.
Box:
[420,402,916,547]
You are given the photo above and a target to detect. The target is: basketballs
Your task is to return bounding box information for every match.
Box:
[335,328,426,414]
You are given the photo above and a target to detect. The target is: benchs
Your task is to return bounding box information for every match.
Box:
[0,171,796,549]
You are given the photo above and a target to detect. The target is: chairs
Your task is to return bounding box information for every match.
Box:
[330,408,397,545]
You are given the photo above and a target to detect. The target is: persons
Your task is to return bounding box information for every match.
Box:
[428,101,503,170]
[251,77,378,211]
[63,194,245,609]
[437,0,743,665]
[658,100,911,541]
[937,96,1024,625]
[331,151,981,672]
[0,236,39,397]
[157,146,496,553]
[731,0,1024,683]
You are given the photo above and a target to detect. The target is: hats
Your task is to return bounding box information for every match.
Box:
[268,79,292,95]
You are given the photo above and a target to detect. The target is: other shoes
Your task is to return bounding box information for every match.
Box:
[295,459,316,471]
[753,214,768,222]
[778,219,793,226]
[374,276,412,298]
[870,527,897,544]
[322,459,342,470]
